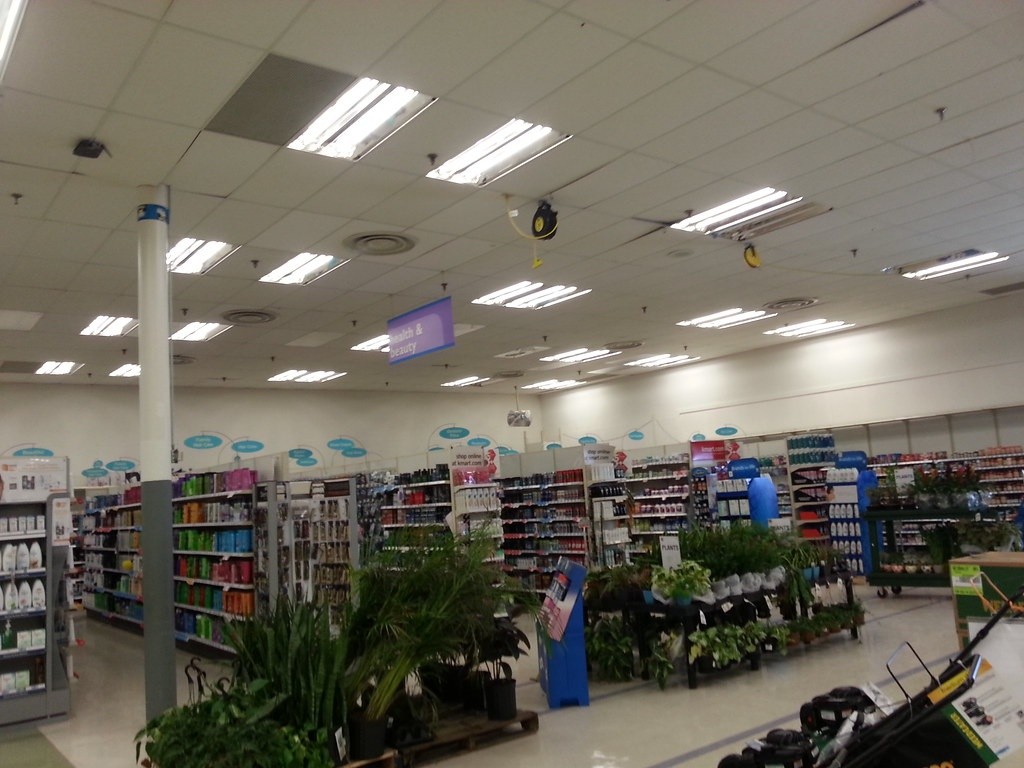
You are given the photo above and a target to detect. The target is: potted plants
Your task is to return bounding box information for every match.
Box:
[794,541,819,581]
[813,612,830,637]
[362,521,498,750]
[761,622,787,653]
[586,570,609,591]
[835,608,853,629]
[346,576,470,760]
[308,597,349,767]
[826,548,848,575]
[829,614,841,634]
[786,622,800,648]
[879,463,1024,575]
[638,518,793,608]
[800,618,816,641]
[688,627,722,674]
[477,617,529,722]
[851,601,866,624]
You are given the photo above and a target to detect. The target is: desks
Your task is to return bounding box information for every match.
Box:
[948,551,1024,657]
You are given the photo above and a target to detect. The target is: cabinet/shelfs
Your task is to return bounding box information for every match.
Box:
[867,453,1024,546]
[784,434,837,547]
[447,444,509,619]
[494,442,591,594]
[625,460,692,554]
[581,441,634,569]
[380,480,452,572]
[716,458,779,528]
[0,455,71,724]
[583,596,637,678]
[72,511,84,600]
[861,504,1015,599]
[79,490,256,655]
[55,535,83,699]
[760,464,792,515]
[825,450,884,582]
[624,570,859,690]
[309,478,360,623]
